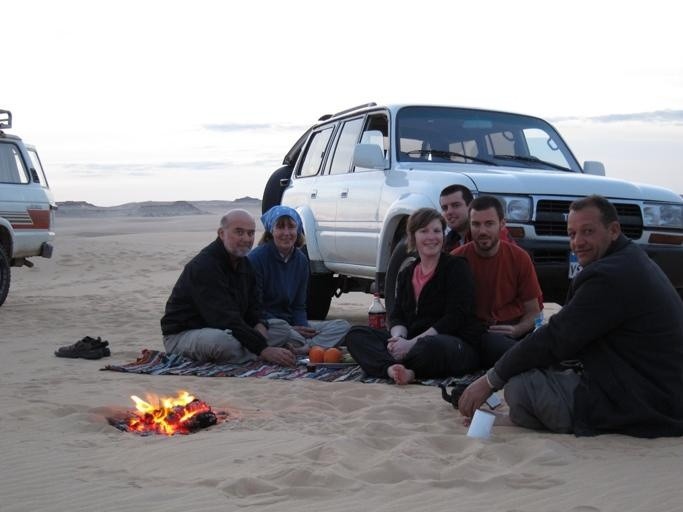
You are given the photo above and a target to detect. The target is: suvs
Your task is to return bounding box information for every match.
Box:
[255,99,682,323]
[0,109,59,310]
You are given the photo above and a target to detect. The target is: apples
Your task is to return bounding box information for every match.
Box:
[308,348,343,363]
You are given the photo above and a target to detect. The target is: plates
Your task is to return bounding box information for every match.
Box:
[299,358,358,368]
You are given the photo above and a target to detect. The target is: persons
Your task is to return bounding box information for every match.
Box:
[159,208,297,368]
[344,207,488,385]
[457,195,683,439]
[446,196,544,336]
[246,205,352,356]
[434,184,516,252]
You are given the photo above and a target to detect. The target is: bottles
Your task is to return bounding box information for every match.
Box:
[533,312,545,327]
[368,293,386,328]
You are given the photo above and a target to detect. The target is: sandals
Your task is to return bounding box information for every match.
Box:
[55,337,110,358]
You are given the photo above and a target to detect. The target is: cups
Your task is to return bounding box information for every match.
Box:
[466,407,496,438]
